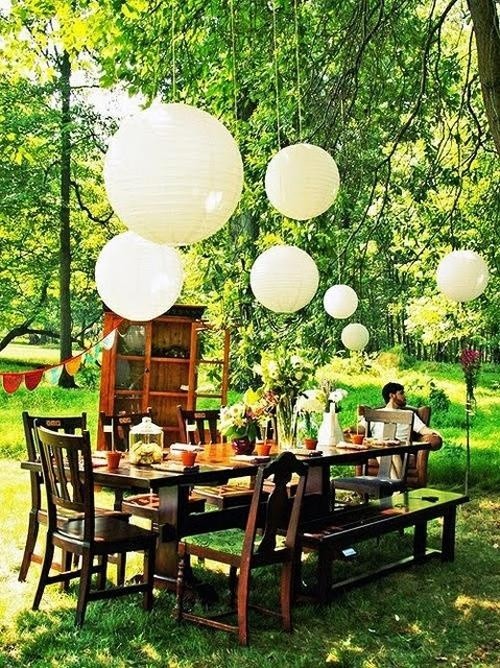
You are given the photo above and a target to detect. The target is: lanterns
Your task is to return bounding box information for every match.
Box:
[341,323,369,350]
[438,250,488,302]
[324,284,358,318]
[251,246,319,313]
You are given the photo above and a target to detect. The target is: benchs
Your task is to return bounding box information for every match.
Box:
[278,485,471,617]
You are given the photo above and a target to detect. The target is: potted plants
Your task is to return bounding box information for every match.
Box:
[296,408,327,451]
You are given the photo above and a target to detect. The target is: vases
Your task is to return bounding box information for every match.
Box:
[318,411,344,445]
[232,436,257,455]
[274,401,296,449]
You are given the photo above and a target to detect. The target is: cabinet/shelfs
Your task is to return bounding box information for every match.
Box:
[95,300,231,453]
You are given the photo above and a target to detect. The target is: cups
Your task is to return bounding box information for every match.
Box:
[181,451,198,466]
[105,452,121,473]
[256,444,272,456]
[351,435,365,445]
[304,439,318,450]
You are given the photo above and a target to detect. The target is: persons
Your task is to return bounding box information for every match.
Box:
[342,381,443,495]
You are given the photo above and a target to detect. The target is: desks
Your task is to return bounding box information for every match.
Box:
[19,439,433,624]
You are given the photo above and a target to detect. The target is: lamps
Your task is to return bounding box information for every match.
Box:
[433,247,488,306]
[338,321,372,350]
[320,282,361,321]
[245,242,322,315]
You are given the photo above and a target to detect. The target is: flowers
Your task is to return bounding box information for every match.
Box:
[217,401,263,443]
[243,353,316,405]
[456,347,485,406]
[295,378,350,414]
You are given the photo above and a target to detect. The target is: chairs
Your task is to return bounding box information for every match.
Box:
[173,402,265,577]
[357,400,435,493]
[28,415,159,629]
[175,448,313,652]
[263,476,300,498]
[15,408,135,593]
[331,403,417,548]
[99,407,212,567]
